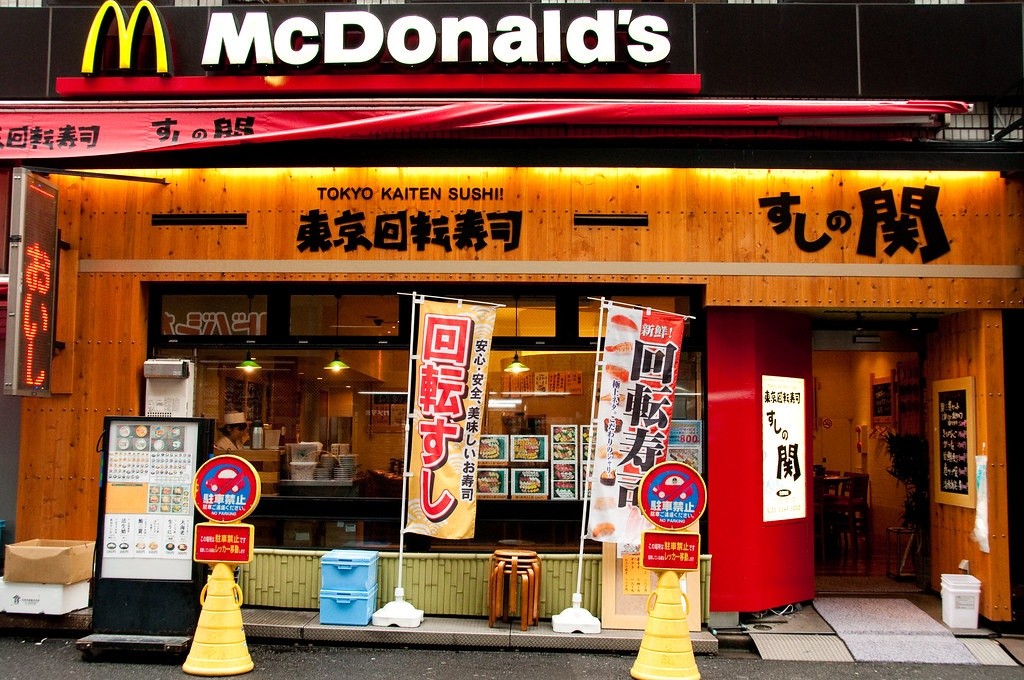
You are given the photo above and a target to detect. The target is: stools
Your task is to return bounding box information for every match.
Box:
[487,549,542,630]
[887,527,922,582]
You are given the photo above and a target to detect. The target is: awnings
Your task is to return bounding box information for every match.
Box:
[0,99,975,164]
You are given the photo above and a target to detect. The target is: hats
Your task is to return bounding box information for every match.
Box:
[224,412,246,425]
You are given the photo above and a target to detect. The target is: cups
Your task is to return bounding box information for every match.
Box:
[265,430,280,450]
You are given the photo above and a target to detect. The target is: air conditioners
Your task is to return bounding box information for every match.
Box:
[504,300,529,373]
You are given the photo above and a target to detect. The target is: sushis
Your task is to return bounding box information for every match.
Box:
[593,523,614,537]
[611,315,638,331]
[605,418,623,433]
[477,428,596,500]
[595,498,614,510]
[600,394,625,404]
[605,342,633,353]
[605,366,629,381]
[601,471,616,486]
[599,448,623,458]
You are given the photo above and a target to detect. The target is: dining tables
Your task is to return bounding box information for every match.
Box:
[816,474,850,496]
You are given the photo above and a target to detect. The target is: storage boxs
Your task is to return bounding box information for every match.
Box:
[0,576,90,616]
[941,573,982,628]
[318,582,379,625]
[4,538,96,585]
[320,549,379,592]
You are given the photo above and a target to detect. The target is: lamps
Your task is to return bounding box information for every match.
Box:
[322,293,350,371]
[237,290,262,370]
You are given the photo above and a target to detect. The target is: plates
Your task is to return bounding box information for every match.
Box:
[170,426,182,437]
[554,481,575,498]
[518,471,542,493]
[516,437,540,460]
[153,440,164,451]
[118,426,130,437]
[553,464,575,480]
[477,471,502,494]
[135,425,148,437]
[553,444,575,461]
[315,453,358,480]
[153,427,165,438]
[553,427,575,443]
[479,438,501,459]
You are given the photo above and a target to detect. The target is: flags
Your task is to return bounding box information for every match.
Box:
[585,304,683,545]
[406,297,498,540]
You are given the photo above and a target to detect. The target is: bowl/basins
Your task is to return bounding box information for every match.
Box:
[135,438,147,450]
[169,439,183,450]
[118,438,130,450]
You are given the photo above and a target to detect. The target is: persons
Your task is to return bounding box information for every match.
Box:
[216,411,247,450]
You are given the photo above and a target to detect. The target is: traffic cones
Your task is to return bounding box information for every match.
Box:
[179,562,255,676]
[628,570,701,680]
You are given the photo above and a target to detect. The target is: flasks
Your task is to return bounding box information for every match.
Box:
[250,420,263,449]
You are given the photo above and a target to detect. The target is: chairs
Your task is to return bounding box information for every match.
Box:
[816,466,872,565]
